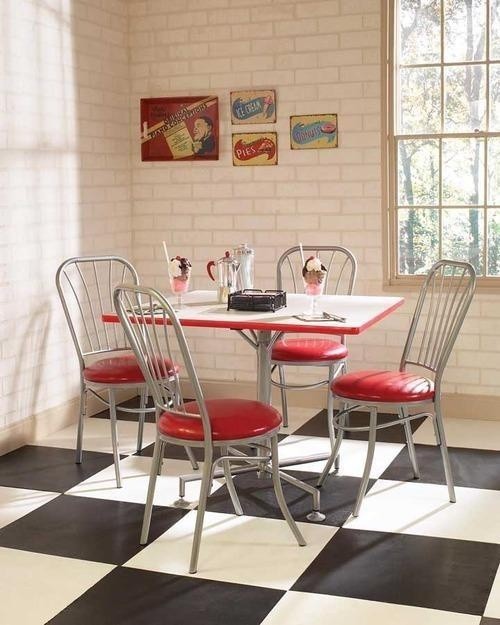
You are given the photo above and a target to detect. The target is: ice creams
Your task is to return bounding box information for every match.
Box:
[169,255,192,294]
[301,255,328,297]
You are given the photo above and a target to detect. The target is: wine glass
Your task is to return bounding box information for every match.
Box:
[167,265,192,314]
[301,270,327,316]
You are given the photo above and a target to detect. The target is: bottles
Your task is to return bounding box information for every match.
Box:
[232,242,255,290]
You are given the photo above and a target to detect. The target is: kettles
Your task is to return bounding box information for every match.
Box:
[207,253,240,304]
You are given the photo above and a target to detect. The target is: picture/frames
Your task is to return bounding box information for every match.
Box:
[140,96,218,161]
